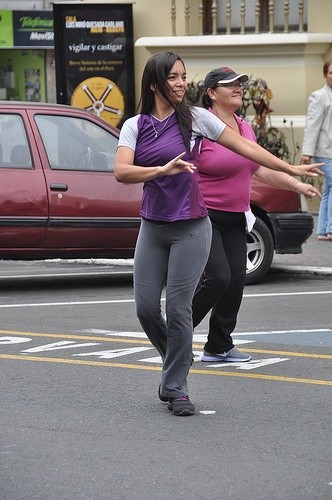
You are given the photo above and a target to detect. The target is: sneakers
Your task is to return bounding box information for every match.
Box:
[167,393,195,416]
[158,354,195,402]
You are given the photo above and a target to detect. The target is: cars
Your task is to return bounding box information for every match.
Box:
[1,100,315,284]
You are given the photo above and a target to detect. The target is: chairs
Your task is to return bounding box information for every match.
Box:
[9,143,29,167]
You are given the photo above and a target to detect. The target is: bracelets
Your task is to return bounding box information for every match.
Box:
[286,176,294,189]
[292,181,300,192]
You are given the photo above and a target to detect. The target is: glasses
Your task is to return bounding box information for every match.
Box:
[216,83,243,89]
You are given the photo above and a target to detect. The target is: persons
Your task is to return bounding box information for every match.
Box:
[112,52,325,415]
[165,66,322,362]
[302,58,332,240]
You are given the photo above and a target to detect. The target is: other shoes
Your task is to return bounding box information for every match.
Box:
[317,232,332,240]
[201,346,252,363]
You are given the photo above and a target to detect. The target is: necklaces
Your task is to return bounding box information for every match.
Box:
[151,110,173,138]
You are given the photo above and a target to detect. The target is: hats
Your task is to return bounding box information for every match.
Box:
[204,67,249,88]
